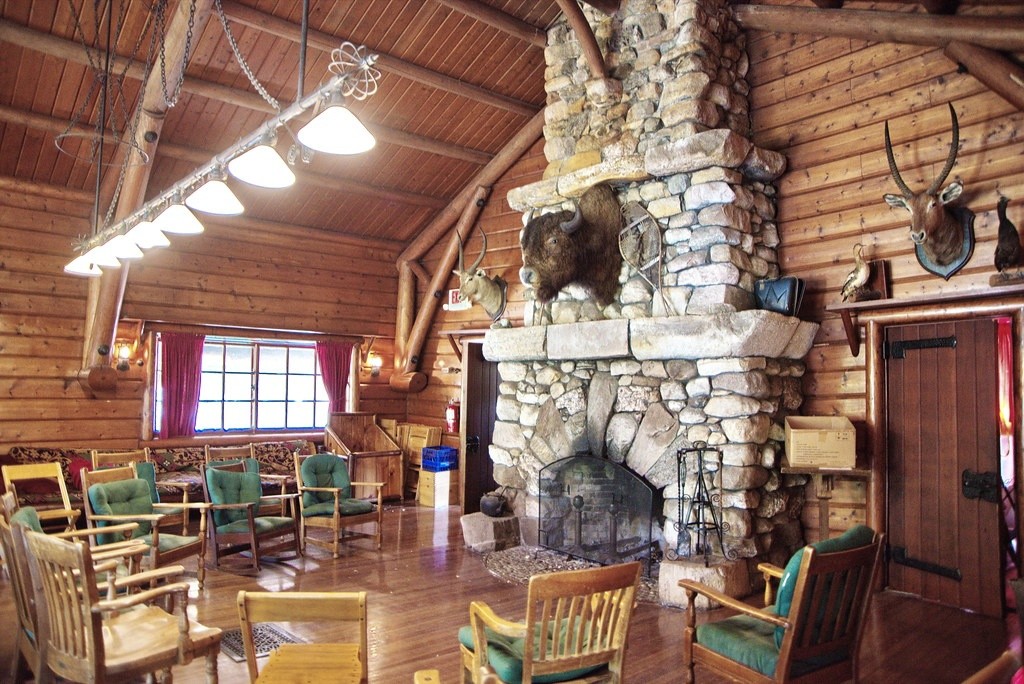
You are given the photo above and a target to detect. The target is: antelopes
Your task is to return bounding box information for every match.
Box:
[882,100,965,268]
[451,224,508,324]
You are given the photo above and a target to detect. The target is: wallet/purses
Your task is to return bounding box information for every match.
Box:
[755,275,806,316]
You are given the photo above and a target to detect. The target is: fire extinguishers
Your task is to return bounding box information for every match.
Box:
[446,398,460,433]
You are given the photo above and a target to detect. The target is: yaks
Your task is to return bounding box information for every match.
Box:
[517,181,627,309]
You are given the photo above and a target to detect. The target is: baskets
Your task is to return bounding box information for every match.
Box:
[423,446,458,471]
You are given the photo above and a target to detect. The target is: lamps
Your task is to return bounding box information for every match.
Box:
[151,194,205,235]
[185,169,245,216]
[125,213,171,249]
[64,251,102,276]
[296,89,377,156]
[227,134,297,189]
[82,246,121,267]
[117,346,130,370]
[100,233,145,260]
[370,357,382,378]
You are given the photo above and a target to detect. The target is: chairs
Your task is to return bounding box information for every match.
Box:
[457,561,644,684]
[0,440,386,684]
[678,525,886,684]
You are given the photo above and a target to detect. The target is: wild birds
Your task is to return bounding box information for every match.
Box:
[840,243,874,303]
[993,196,1024,273]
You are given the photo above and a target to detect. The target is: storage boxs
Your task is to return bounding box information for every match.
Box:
[784,415,857,469]
[756,275,807,317]
[421,446,458,472]
